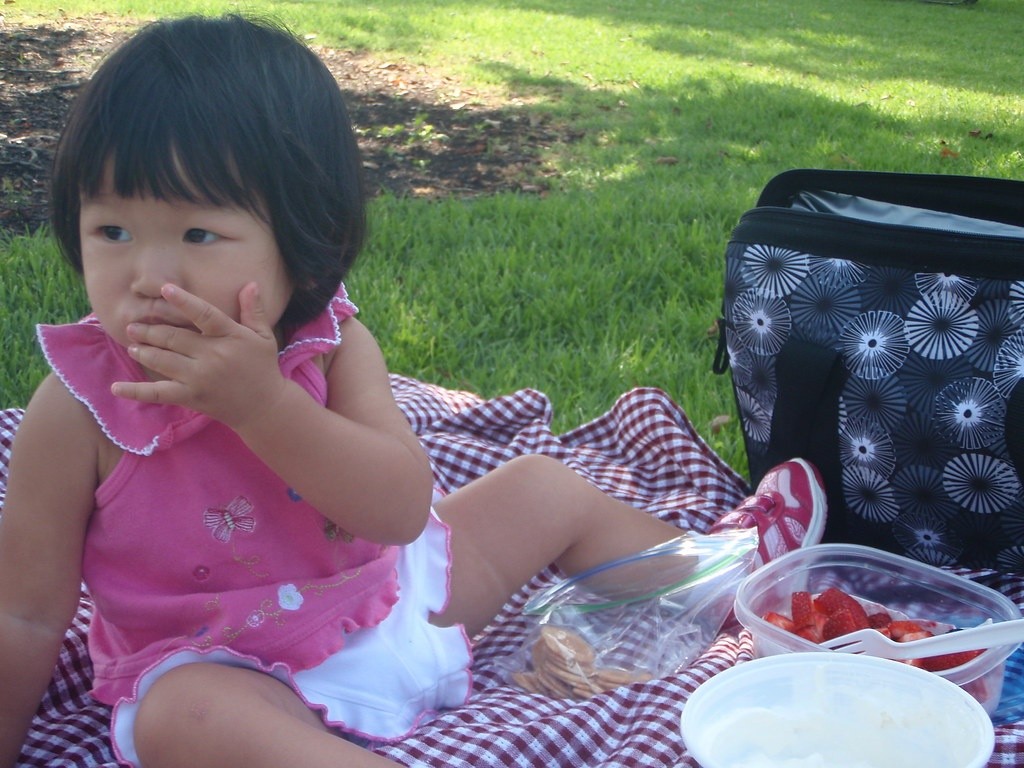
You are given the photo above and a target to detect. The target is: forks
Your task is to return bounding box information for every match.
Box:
[817,620,1024,659]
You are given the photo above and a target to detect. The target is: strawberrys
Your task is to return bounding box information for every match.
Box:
[765,590,992,701]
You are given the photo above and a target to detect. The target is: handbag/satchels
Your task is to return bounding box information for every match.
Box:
[705,166,1024,579]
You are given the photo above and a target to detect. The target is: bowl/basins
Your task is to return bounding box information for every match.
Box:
[683,653,995,768]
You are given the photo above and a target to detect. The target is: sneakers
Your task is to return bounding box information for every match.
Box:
[708,456,830,630]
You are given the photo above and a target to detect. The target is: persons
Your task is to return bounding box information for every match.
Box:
[0,14,829,768]
